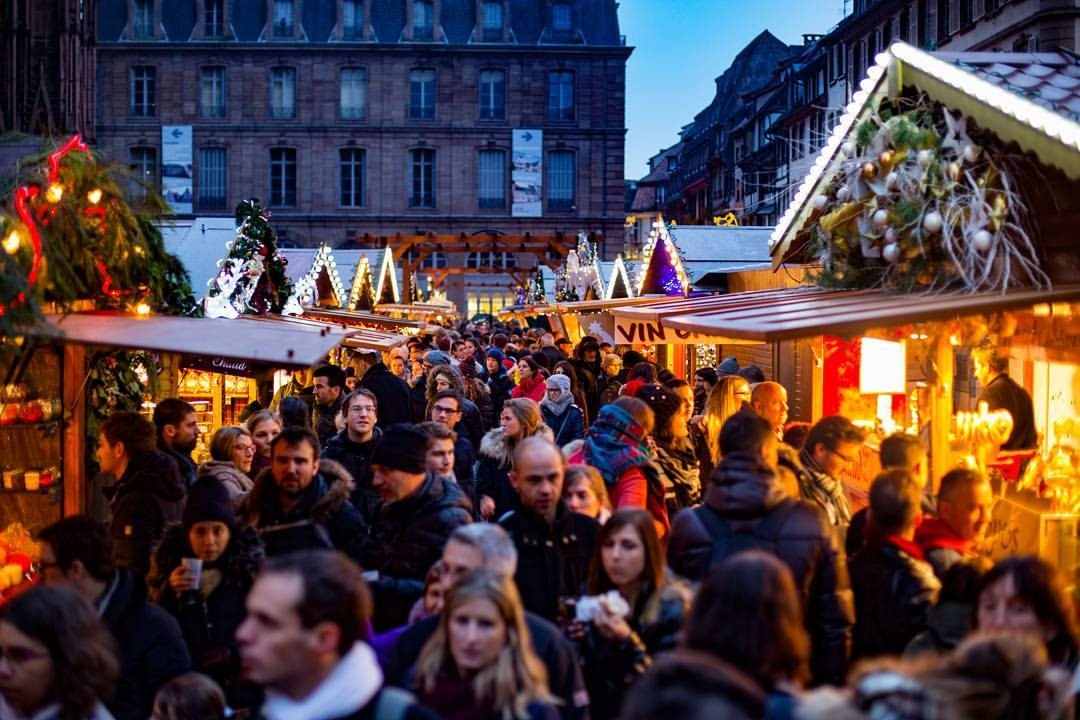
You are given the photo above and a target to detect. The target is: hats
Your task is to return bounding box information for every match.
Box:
[488,348,502,368]
[182,475,237,547]
[546,374,570,402]
[370,423,427,473]
[738,366,764,384]
[716,356,740,377]
[423,350,450,366]
[579,336,602,363]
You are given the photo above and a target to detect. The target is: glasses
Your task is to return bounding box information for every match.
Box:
[823,444,856,465]
[229,444,256,454]
[31,561,60,574]
[0,645,51,664]
[431,406,460,415]
[424,365,432,369]
[347,406,377,415]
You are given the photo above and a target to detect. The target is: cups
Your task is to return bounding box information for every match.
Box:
[182,558,204,591]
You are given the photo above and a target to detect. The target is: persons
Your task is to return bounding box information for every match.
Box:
[0,302,1078,717]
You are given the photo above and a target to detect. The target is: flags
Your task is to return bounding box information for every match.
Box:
[162,125,194,217]
[512,129,544,220]
